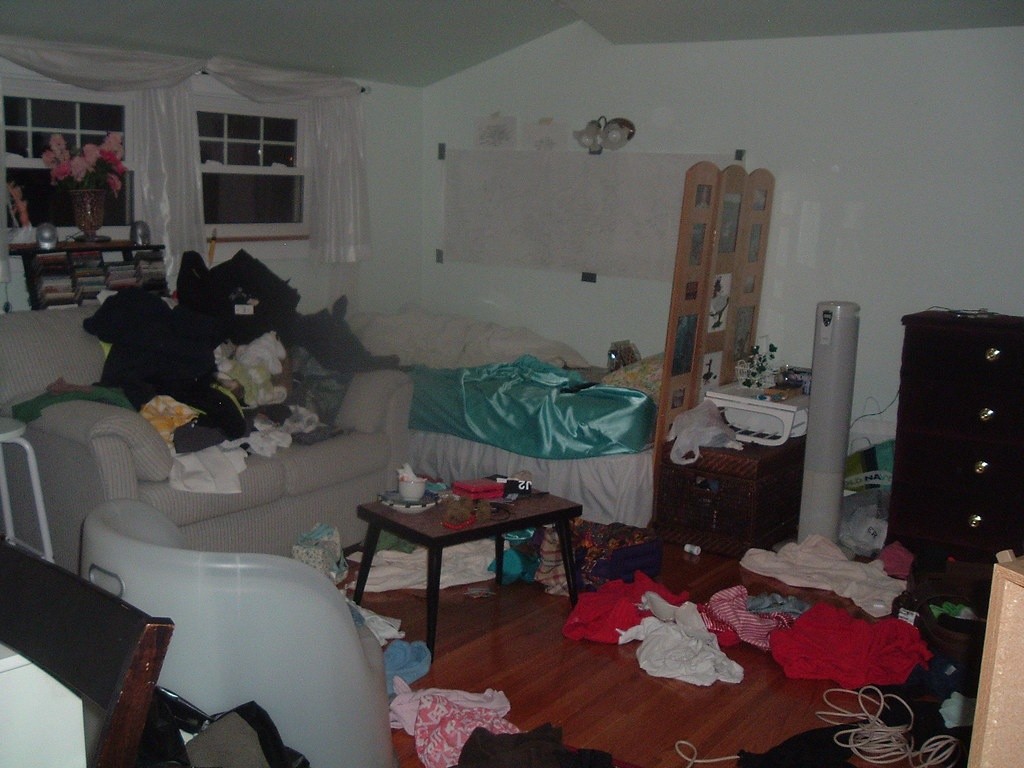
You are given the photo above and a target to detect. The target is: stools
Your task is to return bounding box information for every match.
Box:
[0,416,55,564]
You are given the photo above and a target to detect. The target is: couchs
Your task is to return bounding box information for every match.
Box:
[0,305,413,558]
[80,499,401,768]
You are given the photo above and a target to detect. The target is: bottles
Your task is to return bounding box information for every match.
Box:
[892,589,913,617]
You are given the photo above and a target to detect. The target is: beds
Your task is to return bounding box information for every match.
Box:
[352,300,665,529]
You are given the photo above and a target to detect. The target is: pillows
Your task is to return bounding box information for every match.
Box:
[276,308,379,382]
[843,439,896,492]
[281,342,355,447]
[601,351,665,408]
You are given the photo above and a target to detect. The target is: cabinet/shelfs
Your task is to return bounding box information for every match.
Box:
[967,550,1024,768]
[657,421,806,540]
[19,244,170,311]
[883,311,1024,559]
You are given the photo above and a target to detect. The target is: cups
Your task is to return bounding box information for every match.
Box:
[397,478,427,502]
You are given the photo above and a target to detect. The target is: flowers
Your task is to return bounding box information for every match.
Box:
[42,131,128,198]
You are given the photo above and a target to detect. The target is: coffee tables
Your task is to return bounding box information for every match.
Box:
[353,474,583,664]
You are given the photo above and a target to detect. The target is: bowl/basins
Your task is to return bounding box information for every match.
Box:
[380,494,441,514]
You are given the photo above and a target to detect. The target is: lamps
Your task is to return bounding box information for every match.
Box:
[573,115,636,156]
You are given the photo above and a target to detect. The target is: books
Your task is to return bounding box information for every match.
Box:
[0,250,166,312]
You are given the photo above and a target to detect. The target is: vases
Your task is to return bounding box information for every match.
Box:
[69,189,112,243]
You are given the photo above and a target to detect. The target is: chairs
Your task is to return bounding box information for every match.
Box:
[0,532,176,768]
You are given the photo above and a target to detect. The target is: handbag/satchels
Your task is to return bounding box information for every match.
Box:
[292,522,350,578]
[666,399,736,465]
